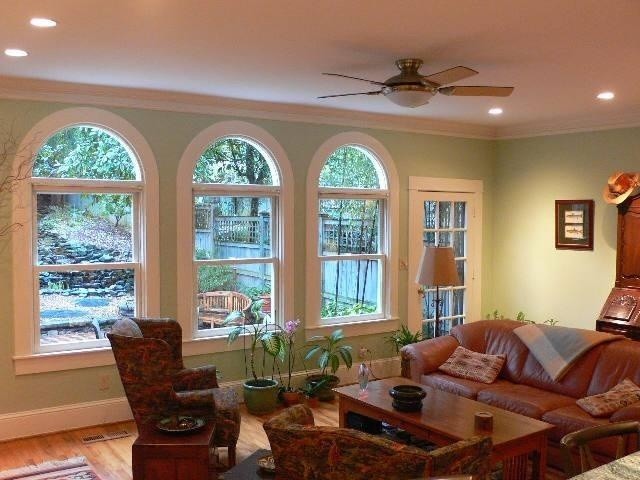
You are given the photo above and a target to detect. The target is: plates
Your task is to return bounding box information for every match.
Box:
[156,416,209,433]
[256,454,276,473]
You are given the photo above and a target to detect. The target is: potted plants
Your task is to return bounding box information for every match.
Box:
[221,309,288,416]
[304,329,352,401]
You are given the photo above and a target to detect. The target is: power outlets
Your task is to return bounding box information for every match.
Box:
[358,349,367,361]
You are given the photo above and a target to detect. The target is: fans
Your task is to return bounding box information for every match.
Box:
[317,59,514,98]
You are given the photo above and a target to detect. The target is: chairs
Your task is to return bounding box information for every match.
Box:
[559,420,640,480]
[196,291,252,328]
[107,318,241,470]
[263,404,496,480]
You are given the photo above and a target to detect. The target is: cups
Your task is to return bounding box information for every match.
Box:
[474,411,494,435]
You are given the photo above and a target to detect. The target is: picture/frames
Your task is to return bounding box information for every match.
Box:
[555,200,593,250]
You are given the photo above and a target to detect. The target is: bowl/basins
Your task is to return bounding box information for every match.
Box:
[389,384,427,406]
[391,398,424,413]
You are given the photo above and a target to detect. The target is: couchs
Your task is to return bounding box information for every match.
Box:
[399,319,640,474]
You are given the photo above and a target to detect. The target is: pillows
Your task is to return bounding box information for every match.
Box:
[575,378,640,417]
[437,346,507,384]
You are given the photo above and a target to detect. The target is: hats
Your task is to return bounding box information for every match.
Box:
[604,170,638,205]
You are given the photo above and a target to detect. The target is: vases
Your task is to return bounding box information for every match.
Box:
[358,361,369,400]
[283,389,302,406]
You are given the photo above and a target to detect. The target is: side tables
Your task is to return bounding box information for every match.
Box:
[132,420,219,480]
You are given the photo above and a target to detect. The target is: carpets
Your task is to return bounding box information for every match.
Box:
[219,449,274,479]
[0,455,102,479]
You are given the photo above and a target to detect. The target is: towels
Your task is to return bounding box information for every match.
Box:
[512,324,627,383]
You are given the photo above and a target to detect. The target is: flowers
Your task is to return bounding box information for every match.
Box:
[284,319,301,391]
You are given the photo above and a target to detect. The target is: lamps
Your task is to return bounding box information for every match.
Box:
[415,245,460,339]
[383,85,436,108]
